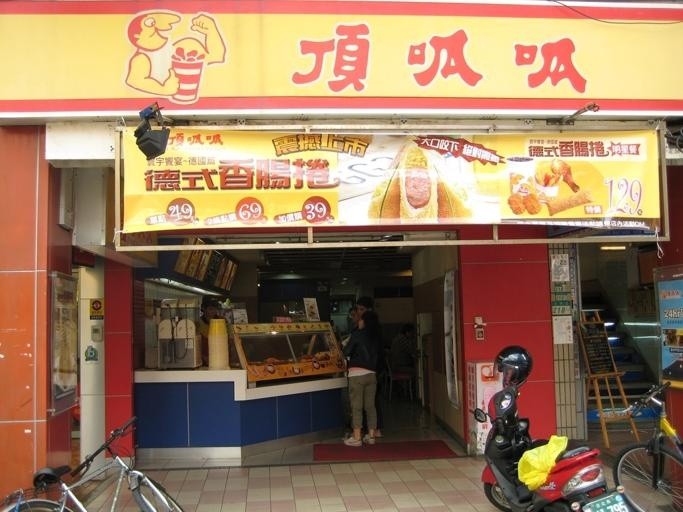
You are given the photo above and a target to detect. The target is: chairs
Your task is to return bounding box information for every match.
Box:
[382,355,415,403]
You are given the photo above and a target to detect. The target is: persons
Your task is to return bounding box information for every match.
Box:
[198,295,228,367]
[341,311,381,448]
[347,306,359,332]
[338,294,384,439]
[394,323,416,375]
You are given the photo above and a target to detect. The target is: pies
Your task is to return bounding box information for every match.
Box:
[368,145,475,224]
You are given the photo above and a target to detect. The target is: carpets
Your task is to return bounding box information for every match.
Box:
[311,440,460,461]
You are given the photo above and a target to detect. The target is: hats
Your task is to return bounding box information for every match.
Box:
[200,294,219,305]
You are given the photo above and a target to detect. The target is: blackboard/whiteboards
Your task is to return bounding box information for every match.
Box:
[578,322,617,377]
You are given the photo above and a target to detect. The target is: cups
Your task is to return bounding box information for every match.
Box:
[506,156,536,197]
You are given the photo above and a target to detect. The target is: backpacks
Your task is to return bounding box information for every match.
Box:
[362,330,391,368]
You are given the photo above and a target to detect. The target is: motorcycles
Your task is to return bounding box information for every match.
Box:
[474,345,631,512]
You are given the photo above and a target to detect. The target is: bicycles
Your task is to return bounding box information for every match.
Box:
[613,381,683,512]
[1,416,185,512]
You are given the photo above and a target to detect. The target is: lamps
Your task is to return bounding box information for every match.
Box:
[133,103,176,161]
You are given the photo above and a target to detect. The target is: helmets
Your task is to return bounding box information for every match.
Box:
[492,344,533,387]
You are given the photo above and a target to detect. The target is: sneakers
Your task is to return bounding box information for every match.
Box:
[342,436,363,446]
[362,428,382,445]
